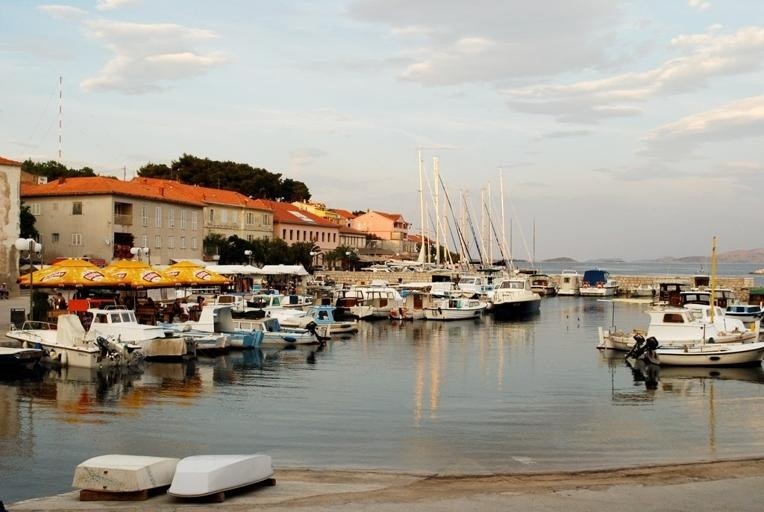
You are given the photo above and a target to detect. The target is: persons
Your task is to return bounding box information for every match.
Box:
[55,293,66,309]
[162,296,209,323]
[0,283,9,300]
[325,275,336,286]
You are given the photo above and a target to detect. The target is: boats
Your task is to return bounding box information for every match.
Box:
[0,367,45,389]
[658,360,763,386]
[45,367,121,407]
[597,279,764,367]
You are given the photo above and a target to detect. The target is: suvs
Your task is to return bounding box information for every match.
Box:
[312,264,329,272]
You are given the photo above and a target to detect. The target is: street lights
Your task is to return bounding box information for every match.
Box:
[243,248,256,269]
[129,244,153,268]
[308,250,320,269]
[344,251,352,271]
[11,235,46,335]
